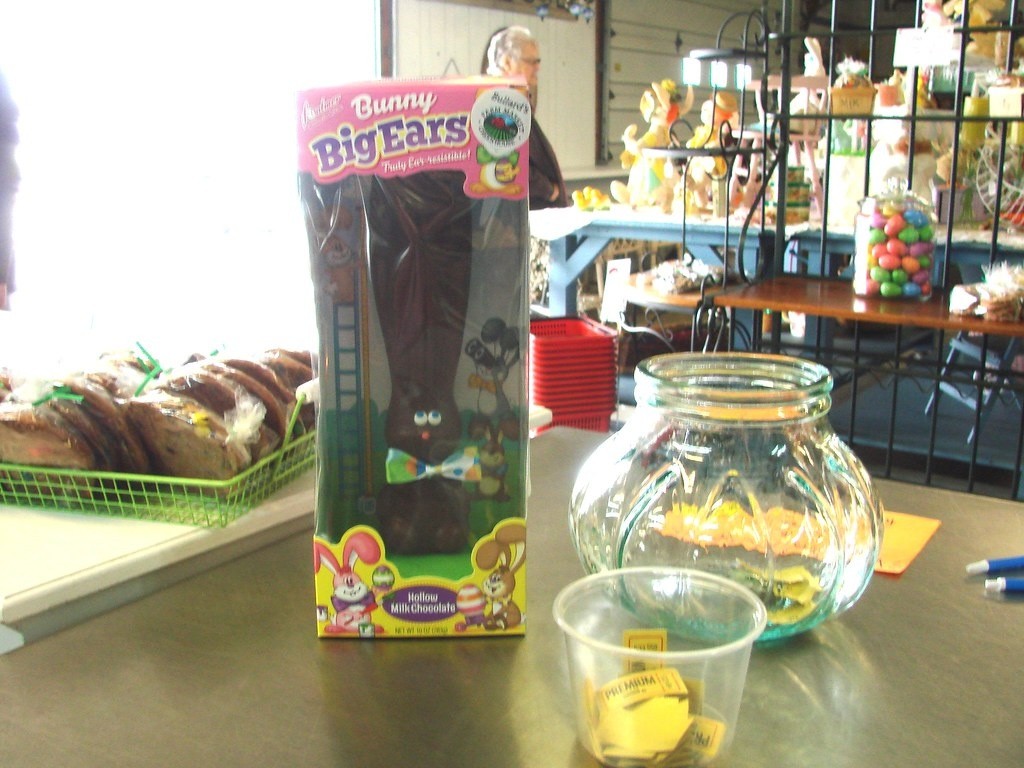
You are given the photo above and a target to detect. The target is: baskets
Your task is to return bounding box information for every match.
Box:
[0,393,317,531]
[527,313,618,434]
[830,72,878,115]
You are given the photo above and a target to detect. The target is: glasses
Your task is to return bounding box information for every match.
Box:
[508,52,540,66]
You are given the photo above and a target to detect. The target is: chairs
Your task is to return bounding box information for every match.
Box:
[925,329,1023,445]
[724,76,830,216]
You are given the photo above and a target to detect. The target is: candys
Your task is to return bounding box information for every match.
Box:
[854,200,937,300]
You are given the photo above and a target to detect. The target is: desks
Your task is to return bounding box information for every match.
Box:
[0,426,1024,768]
[786,225,1024,371]
[626,270,1024,353]
[548,205,807,351]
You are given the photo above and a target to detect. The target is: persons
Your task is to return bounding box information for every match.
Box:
[487,27,568,210]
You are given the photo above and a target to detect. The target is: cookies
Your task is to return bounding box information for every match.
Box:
[0,348,317,505]
[950,274,1024,322]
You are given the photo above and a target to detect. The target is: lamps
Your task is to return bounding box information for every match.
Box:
[536,0,595,24]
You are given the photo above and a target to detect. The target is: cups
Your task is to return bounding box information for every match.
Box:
[551,564,769,768]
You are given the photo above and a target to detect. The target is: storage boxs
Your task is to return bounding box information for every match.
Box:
[528,316,617,436]
[296,78,532,640]
